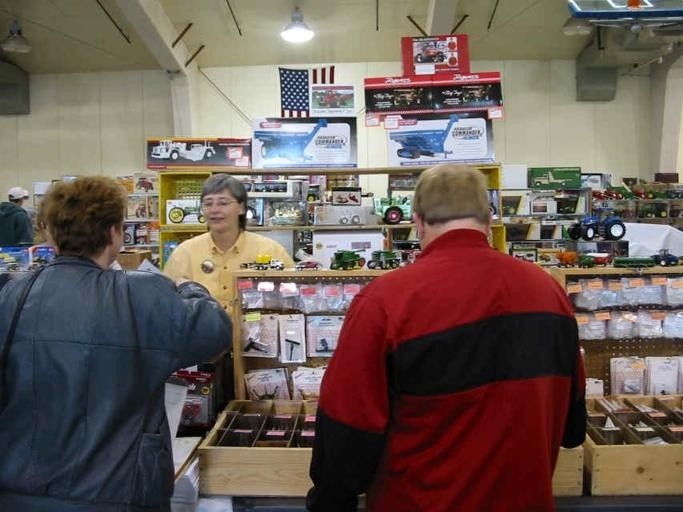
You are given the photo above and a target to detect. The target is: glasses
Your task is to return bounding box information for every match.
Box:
[199,198,240,208]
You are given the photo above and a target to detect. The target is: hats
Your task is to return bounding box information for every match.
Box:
[8,186,30,201]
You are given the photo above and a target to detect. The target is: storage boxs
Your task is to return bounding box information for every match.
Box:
[550,444,584,498]
[582,395,683,497]
[196,398,318,499]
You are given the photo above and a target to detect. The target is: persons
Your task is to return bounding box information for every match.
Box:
[305,164,586,512]
[0,176,233,512]
[0,186,33,245]
[162,173,296,401]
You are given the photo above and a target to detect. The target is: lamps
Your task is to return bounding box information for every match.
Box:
[279,6,315,44]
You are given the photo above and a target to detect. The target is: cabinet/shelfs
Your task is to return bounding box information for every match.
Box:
[157,161,508,275]
